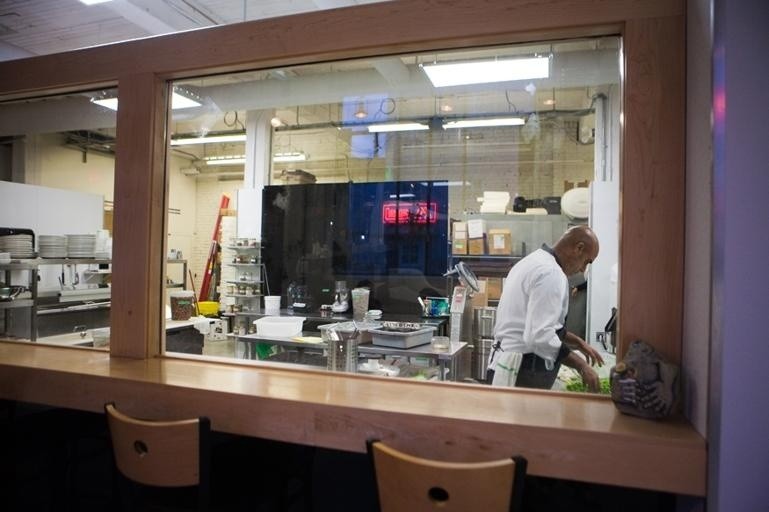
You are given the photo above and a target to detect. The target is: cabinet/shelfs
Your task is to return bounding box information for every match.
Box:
[1,249,219,355]
[222,239,264,335]
[446,241,525,351]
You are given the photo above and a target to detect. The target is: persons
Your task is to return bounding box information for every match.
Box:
[486,223,607,394]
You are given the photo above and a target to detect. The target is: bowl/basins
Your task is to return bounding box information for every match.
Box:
[0,286,24,302]
[196,300,220,315]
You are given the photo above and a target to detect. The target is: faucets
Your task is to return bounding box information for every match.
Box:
[72,323,88,338]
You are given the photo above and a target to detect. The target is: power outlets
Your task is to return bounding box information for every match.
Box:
[595,331,607,343]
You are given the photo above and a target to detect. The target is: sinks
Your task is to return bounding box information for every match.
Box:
[38,330,95,347]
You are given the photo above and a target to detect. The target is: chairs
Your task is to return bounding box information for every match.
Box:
[365,434,528,511]
[102,398,222,511]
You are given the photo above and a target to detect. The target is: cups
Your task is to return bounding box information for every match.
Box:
[431,335,450,354]
[352,287,370,317]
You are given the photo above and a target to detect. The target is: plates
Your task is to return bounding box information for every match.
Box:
[370,309,382,315]
[369,315,382,319]
[0,229,111,266]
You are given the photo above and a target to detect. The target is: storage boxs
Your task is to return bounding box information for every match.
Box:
[471,273,503,308]
[452,220,512,257]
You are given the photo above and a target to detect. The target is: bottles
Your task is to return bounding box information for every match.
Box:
[286,279,350,317]
[169,248,183,259]
[512,190,527,212]
[222,237,257,335]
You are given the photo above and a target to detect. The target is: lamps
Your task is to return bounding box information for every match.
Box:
[170,130,246,146]
[90,85,205,115]
[204,150,307,166]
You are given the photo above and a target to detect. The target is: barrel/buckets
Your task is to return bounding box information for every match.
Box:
[196,300,220,317]
[169,289,195,321]
